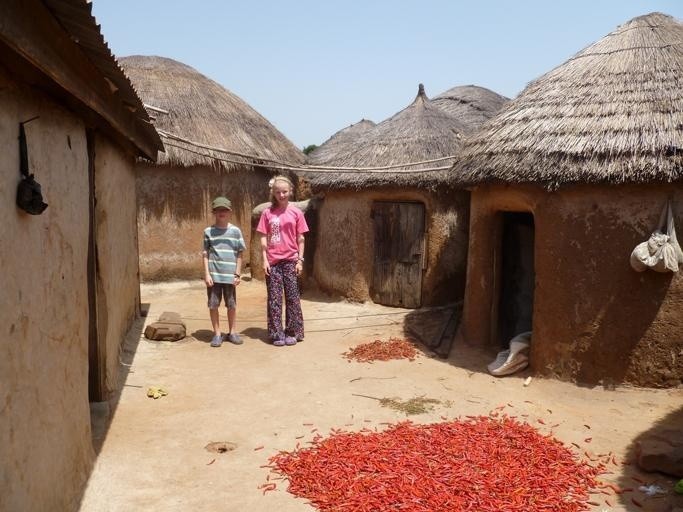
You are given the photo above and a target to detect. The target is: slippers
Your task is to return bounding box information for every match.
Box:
[210,334,243,347]
[271,334,297,346]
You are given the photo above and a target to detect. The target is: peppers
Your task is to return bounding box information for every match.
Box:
[206,458,215,465]
[255,400,643,512]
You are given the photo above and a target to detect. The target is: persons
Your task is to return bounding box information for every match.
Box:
[201,196,247,347]
[255,176,309,346]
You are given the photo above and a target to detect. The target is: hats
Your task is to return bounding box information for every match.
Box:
[213,196,232,210]
